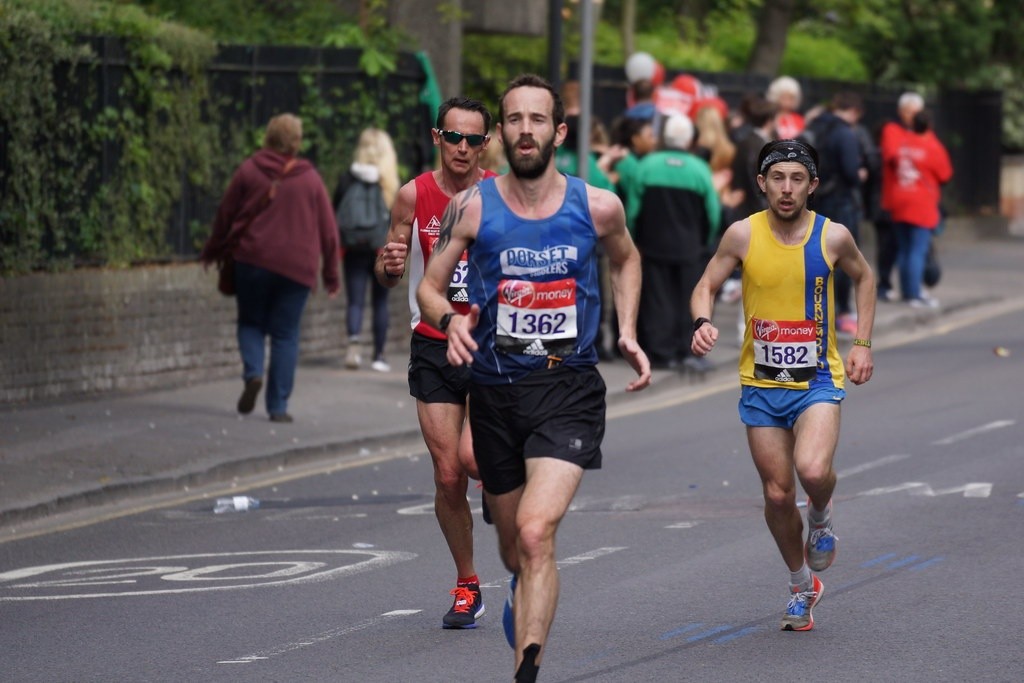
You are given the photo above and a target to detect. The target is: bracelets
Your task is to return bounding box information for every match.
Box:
[384,265,405,279]
[854,338,872,348]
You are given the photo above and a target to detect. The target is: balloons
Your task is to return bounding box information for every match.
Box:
[626,53,727,123]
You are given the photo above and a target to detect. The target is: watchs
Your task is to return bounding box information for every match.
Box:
[439,312,458,334]
[692,317,712,332]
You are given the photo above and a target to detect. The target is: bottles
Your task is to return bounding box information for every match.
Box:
[213,496,259,514]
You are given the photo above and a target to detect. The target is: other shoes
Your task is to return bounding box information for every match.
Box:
[237,377,261,414]
[372,355,391,371]
[344,341,360,370]
[502,574,518,650]
[270,412,293,424]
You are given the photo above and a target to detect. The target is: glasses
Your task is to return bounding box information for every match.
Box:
[435,127,489,148]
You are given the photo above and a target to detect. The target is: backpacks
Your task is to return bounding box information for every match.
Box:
[335,181,391,248]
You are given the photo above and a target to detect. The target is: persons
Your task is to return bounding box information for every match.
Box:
[417,74,651,683]
[690,139,877,631]
[200,114,341,424]
[376,95,501,628]
[480,76,952,380]
[332,127,401,373]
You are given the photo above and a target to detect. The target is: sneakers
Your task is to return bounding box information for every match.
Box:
[781,574,826,630]
[440,584,488,630]
[804,496,837,573]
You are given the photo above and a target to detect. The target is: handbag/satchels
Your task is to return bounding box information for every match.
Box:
[218,258,238,295]
